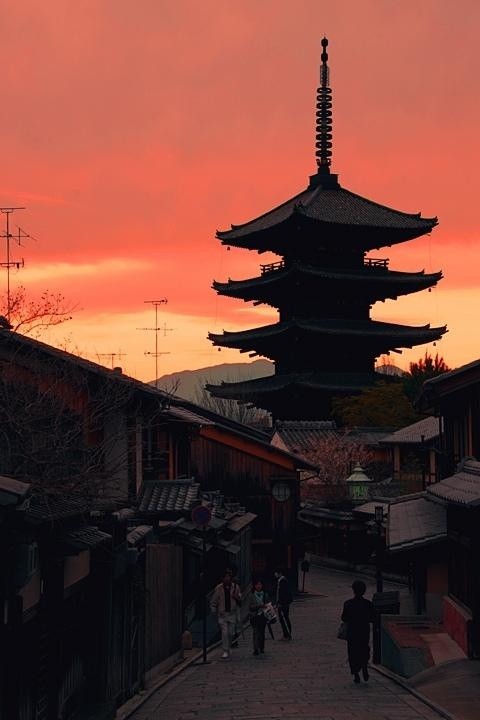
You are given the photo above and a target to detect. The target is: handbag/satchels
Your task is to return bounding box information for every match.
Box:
[335,621,350,640]
[259,602,277,622]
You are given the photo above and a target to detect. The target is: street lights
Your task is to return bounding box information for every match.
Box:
[372,504,386,594]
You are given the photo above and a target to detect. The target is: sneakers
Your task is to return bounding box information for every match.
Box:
[353,674,360,684]
[278,635,293,642]
[220,648,233,659]
[252,645,266,655]
[363,669,370,681]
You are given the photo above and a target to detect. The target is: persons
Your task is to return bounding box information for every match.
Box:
[247,577,271,658]
[339,580,378,685]
[209,570,243,661]
[269,567,295,641]
